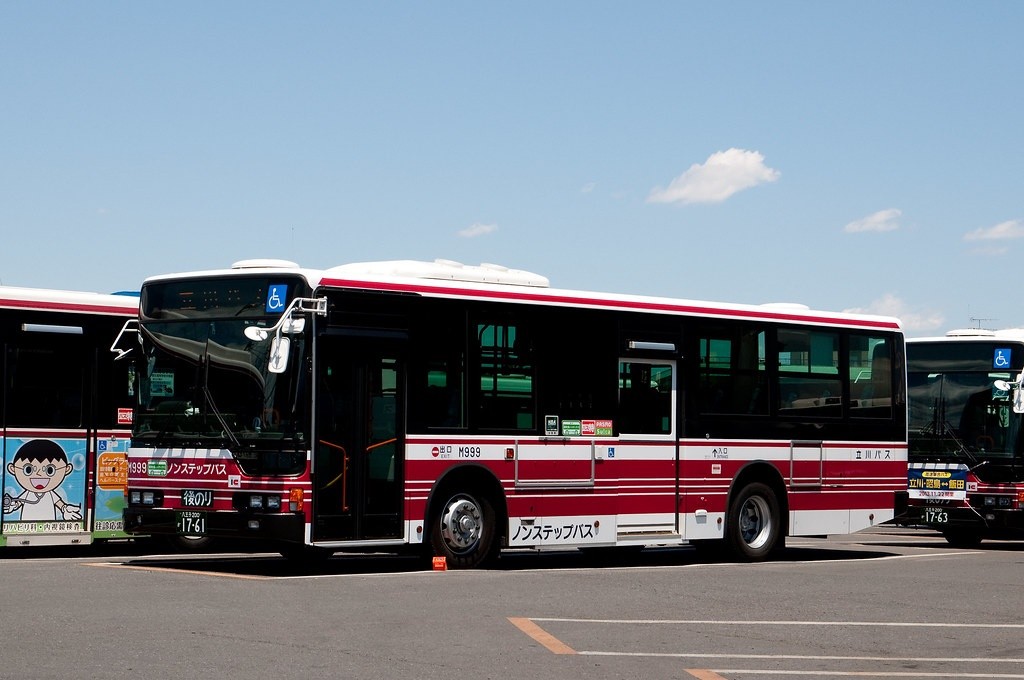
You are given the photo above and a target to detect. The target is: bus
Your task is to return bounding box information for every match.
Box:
[854,326,1024,548]
[107,257,911,569]
[652,353,872,413]
[0,287,659,559]
